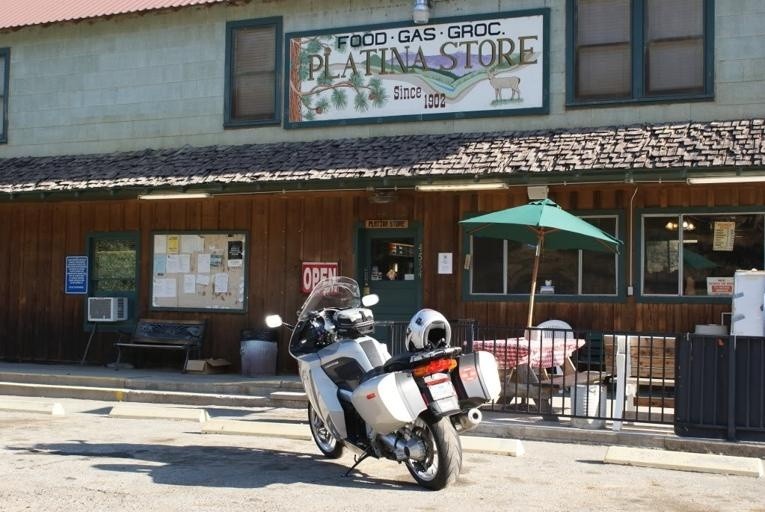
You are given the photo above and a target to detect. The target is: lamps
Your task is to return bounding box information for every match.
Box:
[412,0,431,25]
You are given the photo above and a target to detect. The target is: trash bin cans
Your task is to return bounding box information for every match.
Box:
[240,328,278,378]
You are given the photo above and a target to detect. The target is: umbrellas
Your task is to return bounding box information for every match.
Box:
[454,195,626,326]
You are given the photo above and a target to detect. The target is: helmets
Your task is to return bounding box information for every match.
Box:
[404,307,452,352]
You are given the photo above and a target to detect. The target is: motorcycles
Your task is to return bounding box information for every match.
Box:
[265,276,501,490]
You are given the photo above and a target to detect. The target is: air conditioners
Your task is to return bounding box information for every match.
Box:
[89,295,128,323]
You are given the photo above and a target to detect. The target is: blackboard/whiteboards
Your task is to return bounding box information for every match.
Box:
[148,230,249,313]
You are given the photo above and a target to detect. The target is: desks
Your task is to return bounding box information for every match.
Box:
[464,336,586,423]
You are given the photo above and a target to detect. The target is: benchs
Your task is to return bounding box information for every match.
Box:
[113,318,208,374]
[604,335,678,431]
[535,369,608,422]
